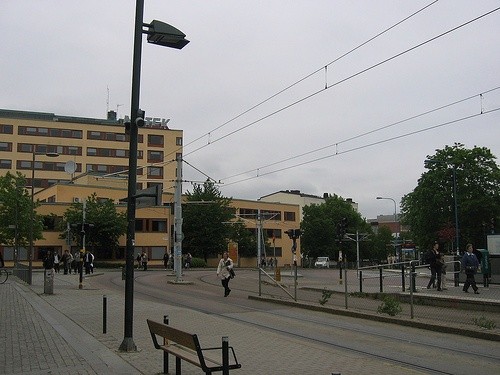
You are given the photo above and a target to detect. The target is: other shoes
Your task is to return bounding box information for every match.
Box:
[227,290,231,295]
[462,289,471,293]
[437,288,442,291]
[474,291,480,294]
[224,294,227,297]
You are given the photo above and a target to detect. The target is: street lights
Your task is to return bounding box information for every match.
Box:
[120,0,190,353]
[377,197,396,261]
[452,144,465,257]
[29,145,59,285]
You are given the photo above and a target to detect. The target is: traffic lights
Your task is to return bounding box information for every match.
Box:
[84,223,94,237]
[341,217,348,239]
[285,229,294,238]
[295,228,302,240]
[71,223,82,235]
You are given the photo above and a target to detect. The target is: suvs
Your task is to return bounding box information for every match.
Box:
[314,256,330,269]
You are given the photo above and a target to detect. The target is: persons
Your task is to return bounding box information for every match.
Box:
[45,250,94,274]
[163,250,192,269]
[426,240,446,292]
[462,243,480,294]
[217,252,233,297]
[135,252,148,270]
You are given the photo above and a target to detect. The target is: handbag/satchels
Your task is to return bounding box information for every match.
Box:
[229,269,236,279]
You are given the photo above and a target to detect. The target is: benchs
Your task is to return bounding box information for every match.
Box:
[146,314,242,375]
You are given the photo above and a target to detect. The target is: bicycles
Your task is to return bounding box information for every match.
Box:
[0,268,9,285]
